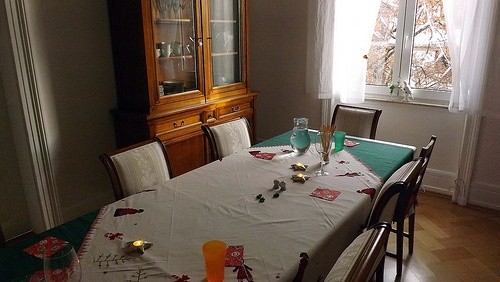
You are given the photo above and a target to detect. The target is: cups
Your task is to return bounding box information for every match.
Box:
[160,44,170,57]
[156,49,160,58]
[43,242,82,282]
[202,240,227,282]
[334,131,346,150]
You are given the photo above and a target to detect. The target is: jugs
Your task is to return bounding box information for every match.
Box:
[290,118,311,154]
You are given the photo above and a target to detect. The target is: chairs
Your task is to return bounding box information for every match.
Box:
[331,104,382,140]
[324,221,387,282]
[201,116,253,162]
[390,134,437,275]
[366,157,423,282]
[99,137,173,202]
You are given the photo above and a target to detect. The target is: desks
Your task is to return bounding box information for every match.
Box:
[0,128,417,282]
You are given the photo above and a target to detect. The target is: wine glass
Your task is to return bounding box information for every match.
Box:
[151,0,187,20]
[315,134,333,176]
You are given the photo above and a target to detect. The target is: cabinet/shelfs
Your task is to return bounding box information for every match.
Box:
[108,0,260,178]
